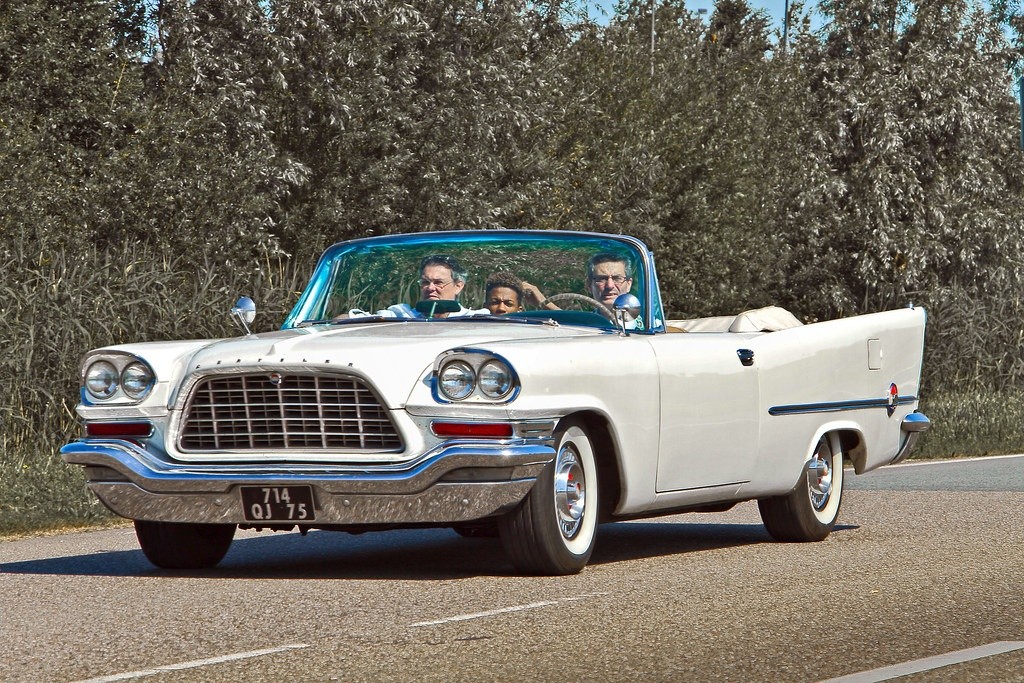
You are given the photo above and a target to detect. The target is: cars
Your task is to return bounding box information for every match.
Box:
[57,229,930,575]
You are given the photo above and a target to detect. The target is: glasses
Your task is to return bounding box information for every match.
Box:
[416,278,455,288]
[592,277,628,286]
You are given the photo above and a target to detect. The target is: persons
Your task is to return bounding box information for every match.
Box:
[334,254,490,319]
[587,251,644,330]
[482,272,561,315]
